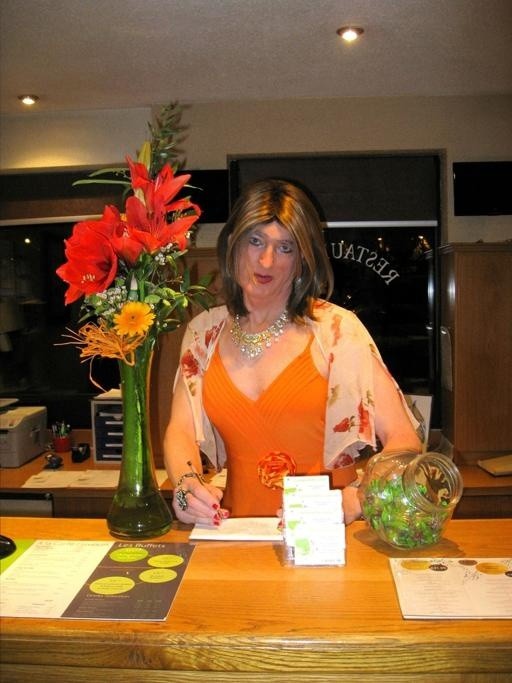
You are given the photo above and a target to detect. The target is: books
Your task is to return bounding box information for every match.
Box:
[389,556,512,620]
[188,514,287,541]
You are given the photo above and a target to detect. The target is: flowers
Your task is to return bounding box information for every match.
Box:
[54,96,225,490]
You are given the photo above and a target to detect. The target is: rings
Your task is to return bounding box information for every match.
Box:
[175,488,190,510]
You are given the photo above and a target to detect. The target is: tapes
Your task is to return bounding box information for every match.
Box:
[78,447,84,453]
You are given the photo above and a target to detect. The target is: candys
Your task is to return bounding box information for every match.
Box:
[362,476,449,549]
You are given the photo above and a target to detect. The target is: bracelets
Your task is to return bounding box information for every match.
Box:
[347,480,360,487]
[176,471,207,486]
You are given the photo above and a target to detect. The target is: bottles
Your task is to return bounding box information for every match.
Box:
[358,447,464,551]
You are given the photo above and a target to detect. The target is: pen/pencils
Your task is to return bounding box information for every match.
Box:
[52,421,70,438]
[187,461,223,519]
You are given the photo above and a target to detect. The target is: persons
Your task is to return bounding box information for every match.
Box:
[155,178,425,530]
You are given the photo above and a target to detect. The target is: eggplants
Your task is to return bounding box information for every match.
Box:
[0,535,16,559]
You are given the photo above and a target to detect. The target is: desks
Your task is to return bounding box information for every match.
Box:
[0,500,511,680]
[0,439,511,517]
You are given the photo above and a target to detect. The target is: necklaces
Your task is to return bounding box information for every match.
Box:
[228,305,290,360]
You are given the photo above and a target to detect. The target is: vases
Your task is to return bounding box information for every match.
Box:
[102,334,173,541]
